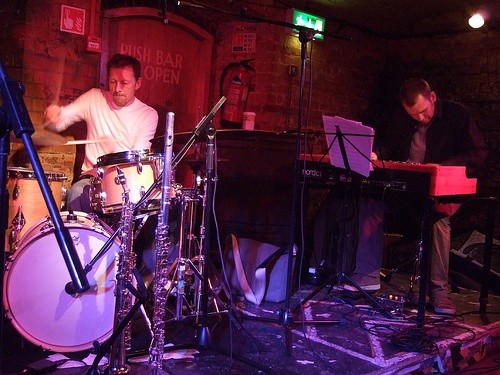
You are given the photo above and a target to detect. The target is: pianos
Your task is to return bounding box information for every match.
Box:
[302,153,476,196]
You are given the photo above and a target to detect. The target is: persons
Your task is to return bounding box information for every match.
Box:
[41,53,161,214]
[339,74,488,318]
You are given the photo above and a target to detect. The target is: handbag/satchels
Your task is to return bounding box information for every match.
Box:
[221,232,298,304]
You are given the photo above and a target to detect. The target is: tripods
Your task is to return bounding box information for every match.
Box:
[165,0,393,356]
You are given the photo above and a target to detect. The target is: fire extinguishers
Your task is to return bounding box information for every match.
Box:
[219,58,255,123]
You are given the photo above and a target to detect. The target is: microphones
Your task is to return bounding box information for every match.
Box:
[161,0,169,24]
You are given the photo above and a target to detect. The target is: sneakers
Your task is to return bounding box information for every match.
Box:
[342,274,381,291]
[429,288,456,314]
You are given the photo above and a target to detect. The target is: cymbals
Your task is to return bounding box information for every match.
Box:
[9,131,67,146]
[149,131,206,144]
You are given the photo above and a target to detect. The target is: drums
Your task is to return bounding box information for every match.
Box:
[2,210,137,353]
[89,148,173,214]
[4,167,64,253]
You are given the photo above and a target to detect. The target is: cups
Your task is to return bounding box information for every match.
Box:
[243,112,255,130]
